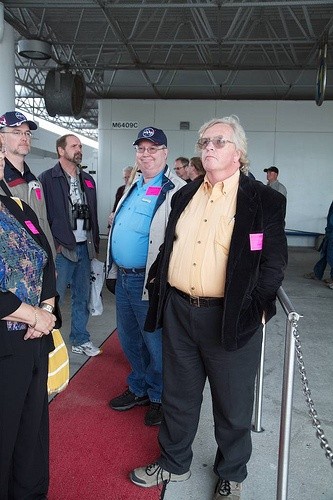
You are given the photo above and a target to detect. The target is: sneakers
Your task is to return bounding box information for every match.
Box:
[213,479,242,500]
[326,281,333,289]
[129,459,191,491]
[72,341,103,356]
[304,272,322,280]
[144,404,161,425]
[109,389,149,410]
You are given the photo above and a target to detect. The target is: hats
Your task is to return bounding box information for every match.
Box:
[264,167,278,175]
[0,111,37,130]
[132,127,168,148]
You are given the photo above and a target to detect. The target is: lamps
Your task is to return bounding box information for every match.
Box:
[18,37,51,60]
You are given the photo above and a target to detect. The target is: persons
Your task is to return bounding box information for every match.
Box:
[173,157,256,184]
[104,126,186,425]
[129,116,289,500]
[39,133,103,357]
[304,201,333,284]
[0,110,59,266]
[0,136,57,500]
[263,165,287,197]
[109,166,134,227]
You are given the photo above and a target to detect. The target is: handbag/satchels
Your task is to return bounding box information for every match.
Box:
[47,328,70,394]
[86,258,105,316]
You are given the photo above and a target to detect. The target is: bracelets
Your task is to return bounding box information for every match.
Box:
[40,303,53,314]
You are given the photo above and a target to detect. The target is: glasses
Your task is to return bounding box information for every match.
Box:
[134,145,167,154]
[0,130,31,138]
[0,145,6,153]
[197,138,235,150]
[173,165,188,171]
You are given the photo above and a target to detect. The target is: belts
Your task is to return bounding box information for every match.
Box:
[165,282,225,308]
[117,266,146,274]
